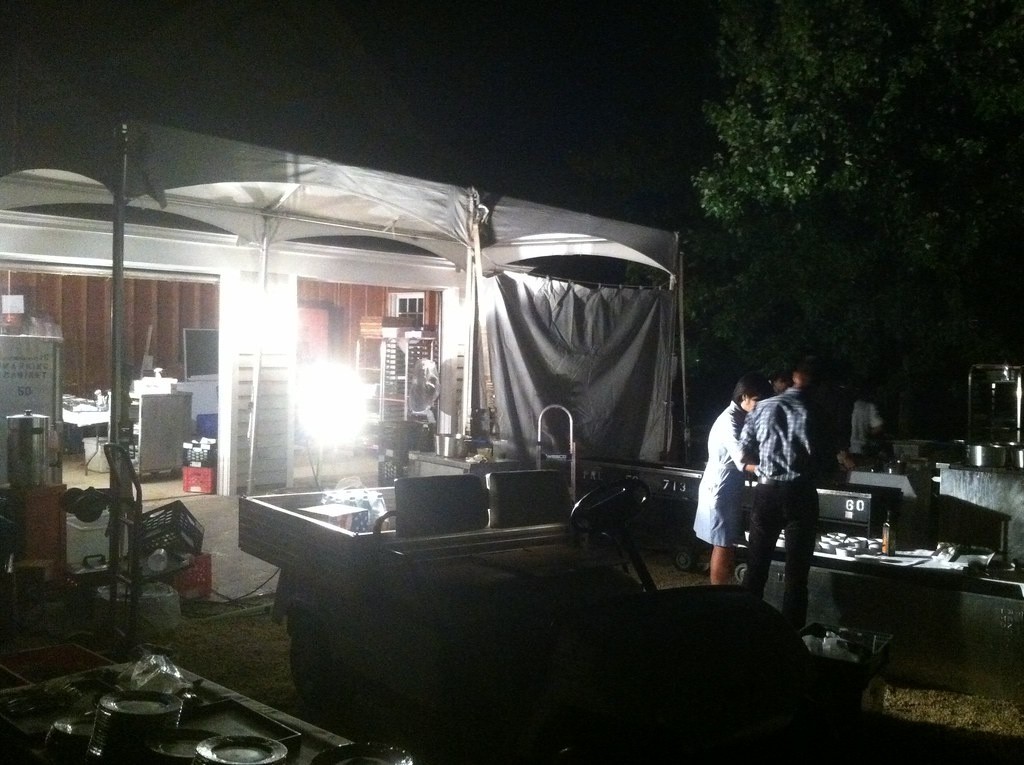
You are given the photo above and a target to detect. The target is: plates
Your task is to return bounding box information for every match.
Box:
[41,689,288,765]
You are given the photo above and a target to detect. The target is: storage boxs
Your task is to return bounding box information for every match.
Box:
[181,439,218,494]
[66,504,213,601]
[382,327,433,338]
[298,503,369,533]
[83,437,111,473]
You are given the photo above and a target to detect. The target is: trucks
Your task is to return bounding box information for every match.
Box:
[238,469,835,765]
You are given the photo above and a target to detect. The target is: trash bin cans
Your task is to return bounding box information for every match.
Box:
[801,622,893,735]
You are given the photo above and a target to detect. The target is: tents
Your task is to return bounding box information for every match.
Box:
[0,84,689,614]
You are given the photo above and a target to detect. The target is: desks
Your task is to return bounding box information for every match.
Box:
[62,408,110,476]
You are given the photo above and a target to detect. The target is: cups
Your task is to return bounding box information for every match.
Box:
[814,531,882,557]
[148,548,168,571]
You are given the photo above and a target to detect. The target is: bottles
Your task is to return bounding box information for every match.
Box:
[881,510,896,557]
[320,488,390,531]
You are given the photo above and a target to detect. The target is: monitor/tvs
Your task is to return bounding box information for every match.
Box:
[180,328,220,381]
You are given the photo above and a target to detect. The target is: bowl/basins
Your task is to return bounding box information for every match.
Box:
[308,741,414,765]
[434,433,473,458]
[953,440,1024,469]
[477,447,492,459]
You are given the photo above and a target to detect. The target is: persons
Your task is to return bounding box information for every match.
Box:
[692,354,840,632]
[847,383,885,471]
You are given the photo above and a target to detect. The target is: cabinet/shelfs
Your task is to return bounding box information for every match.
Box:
[129,391,193,477]
